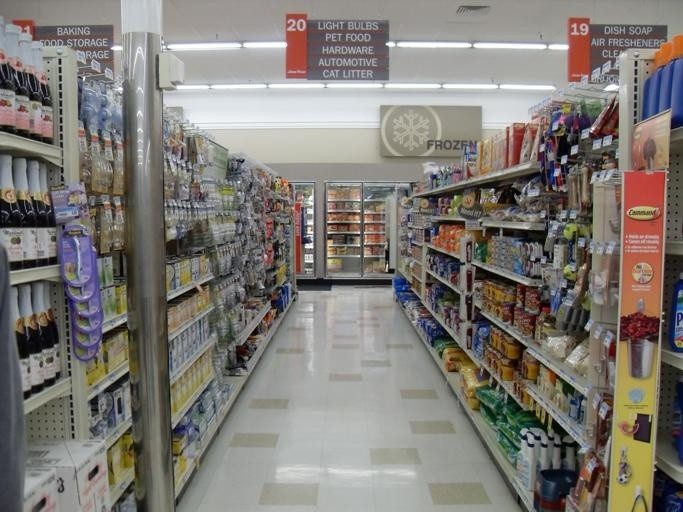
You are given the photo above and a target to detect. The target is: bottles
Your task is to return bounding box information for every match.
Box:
[0,153,59,271]
[10,281,62,401]
[0,16,55,145]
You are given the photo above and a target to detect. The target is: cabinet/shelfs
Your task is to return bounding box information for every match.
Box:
[288,181,411,282]
